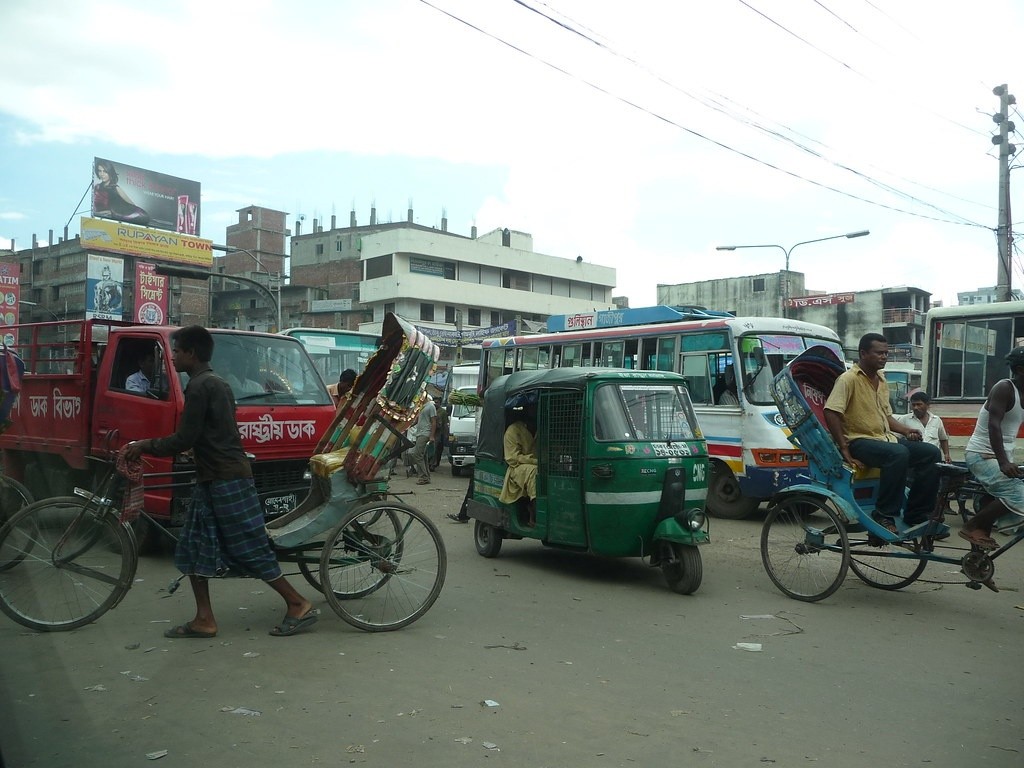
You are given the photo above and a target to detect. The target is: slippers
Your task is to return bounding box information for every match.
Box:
[958,531,1000,548]
[871,509,899,535]
[960,567,999,593]
[164,621,216,638]
[527,522,534,528]
[269,612,318,636]
[903,516,928,527]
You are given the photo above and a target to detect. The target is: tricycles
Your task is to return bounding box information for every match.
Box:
[0,312,452,635]
[758,344,1024,604]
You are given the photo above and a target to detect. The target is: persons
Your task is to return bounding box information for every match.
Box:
[410,392,449,485]
[958,347,1024,593]
[718,365,740,405]
[503,400,538,528]
[95,265,122,312]
[126,344,169,396]
[326,368,357,409]
[125,325,320,638]
[447,387,487,524]
[388,435,400,475]
[178,198,186,231]
[823,334,945,535]
[946,493,975,515]
[223,354,265,399]
[893,392,951,540]
[93,161,143,222]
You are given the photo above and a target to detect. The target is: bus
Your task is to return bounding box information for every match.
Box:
[275,326,382,385]
[880,369,922,422]
[475,302,848,519]
[917,300,1024,501]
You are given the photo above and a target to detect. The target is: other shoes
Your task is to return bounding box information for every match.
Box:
[416,479,431,484]
[447,513,468,523]
[933,532,950,539]
[958,508,975,516]
[390,469,397,475]
[944,508,958,515]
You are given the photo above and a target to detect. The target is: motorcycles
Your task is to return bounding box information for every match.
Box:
[466,367,710,596]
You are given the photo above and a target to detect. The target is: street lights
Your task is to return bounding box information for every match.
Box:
[715,228,871,319]
[153,258,282,333]
[18,300,68,358]
[209,243,272,294]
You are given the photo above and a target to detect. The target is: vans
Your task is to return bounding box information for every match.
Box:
[447,383,478,473]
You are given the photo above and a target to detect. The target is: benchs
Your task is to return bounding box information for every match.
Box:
[309,445,352,484]
[837,444,882,480]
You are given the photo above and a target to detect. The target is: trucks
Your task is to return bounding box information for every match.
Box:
[0,317,339,556]
